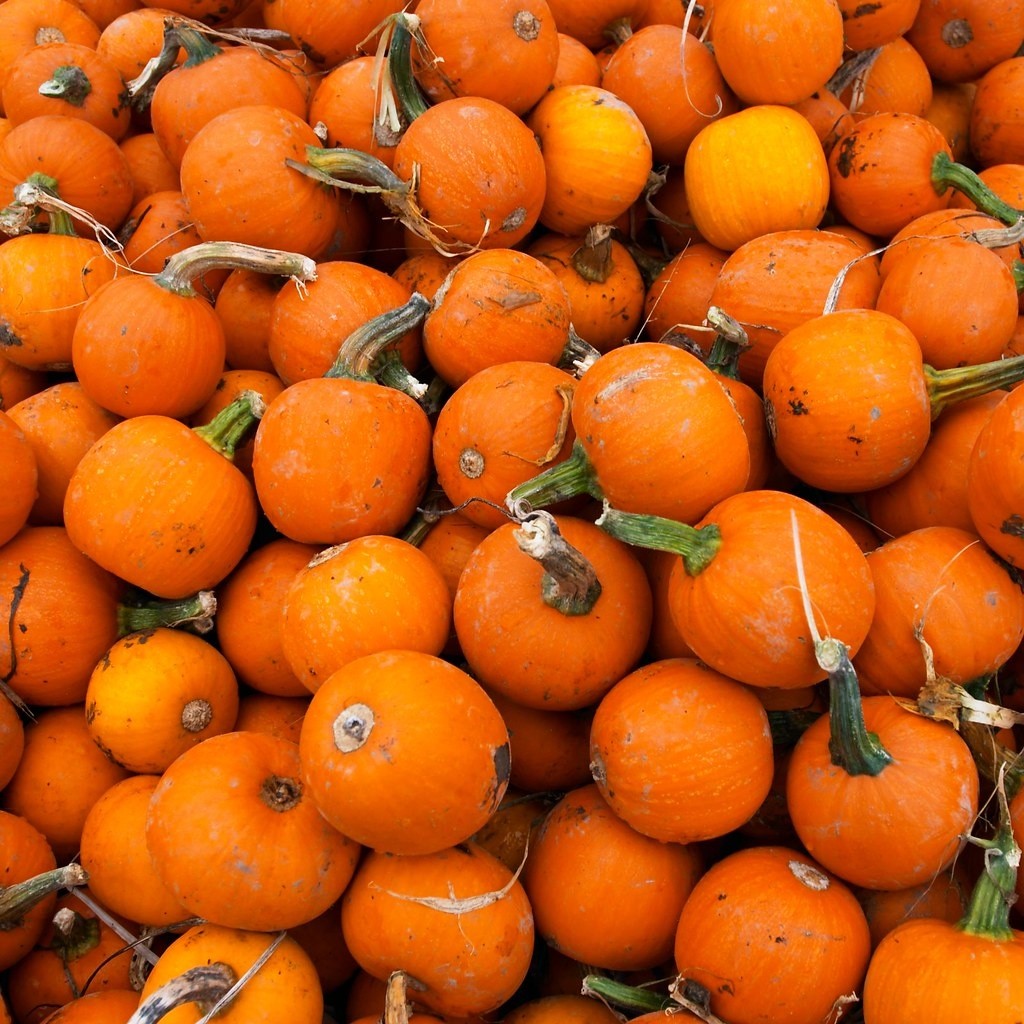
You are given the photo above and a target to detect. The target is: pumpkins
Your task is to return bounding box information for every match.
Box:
[1,0,1022,1024]
